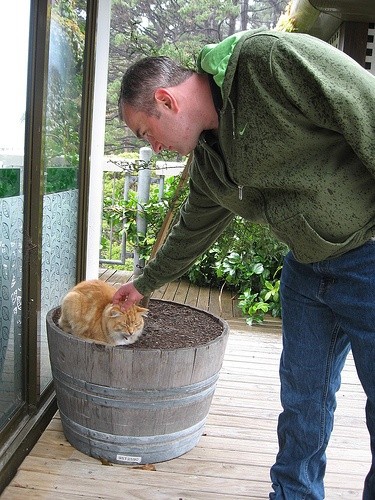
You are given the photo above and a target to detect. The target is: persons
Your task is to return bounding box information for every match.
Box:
[112,23,375,500]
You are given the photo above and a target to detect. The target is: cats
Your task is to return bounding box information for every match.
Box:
[58,279,150,347]
